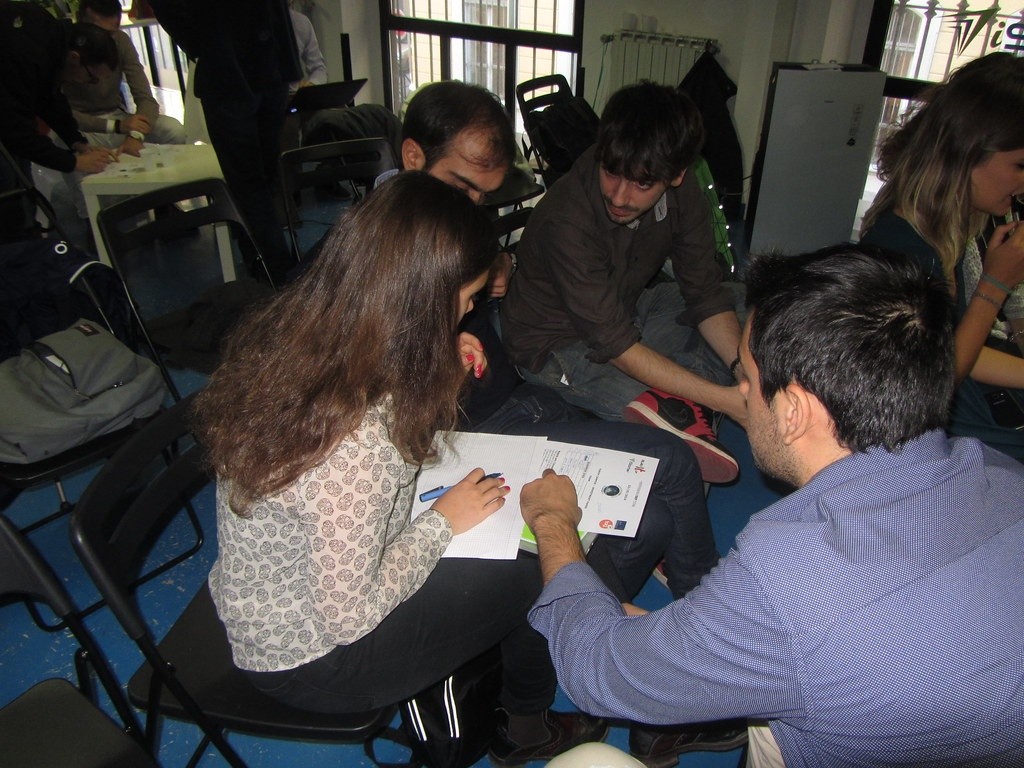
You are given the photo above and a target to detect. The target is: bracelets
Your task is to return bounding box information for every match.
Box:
[728,357,741,381]
[978,274,1014,295]
[970,290,1002,310]
[115,119,122,135]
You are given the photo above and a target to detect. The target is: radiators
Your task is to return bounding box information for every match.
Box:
[607,30,715,98]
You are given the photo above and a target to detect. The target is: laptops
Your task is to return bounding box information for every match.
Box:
[287,78,368,114]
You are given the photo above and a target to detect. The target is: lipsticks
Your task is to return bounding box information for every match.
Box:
[1011,211,1019,223]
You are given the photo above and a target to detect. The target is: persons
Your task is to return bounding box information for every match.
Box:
[290,79,719,599]
[184,170,612,767]
[134,0,329,287]
[492,81,745,488]
[518,239,1024,766]
[854,51,1023,447]
[0,0,184,245]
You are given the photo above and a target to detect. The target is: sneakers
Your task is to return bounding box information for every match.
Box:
[654,560,669,591]
[625,388,740,485]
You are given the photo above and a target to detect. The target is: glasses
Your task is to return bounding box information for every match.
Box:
[83,61,99,85]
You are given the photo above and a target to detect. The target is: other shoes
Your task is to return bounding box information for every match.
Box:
[630,726,748,768]
[154,205,200,237]
[487,708,608,768]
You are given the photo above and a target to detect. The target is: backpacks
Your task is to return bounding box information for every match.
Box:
[0,317,166,464]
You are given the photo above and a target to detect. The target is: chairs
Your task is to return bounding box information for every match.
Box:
[0,75,597,768]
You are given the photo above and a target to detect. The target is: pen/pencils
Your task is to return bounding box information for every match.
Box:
[419,472,504,503]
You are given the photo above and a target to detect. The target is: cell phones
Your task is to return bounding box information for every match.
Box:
[986,389,1024,430]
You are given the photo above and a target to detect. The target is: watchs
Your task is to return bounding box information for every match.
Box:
[128,131,142,139]
